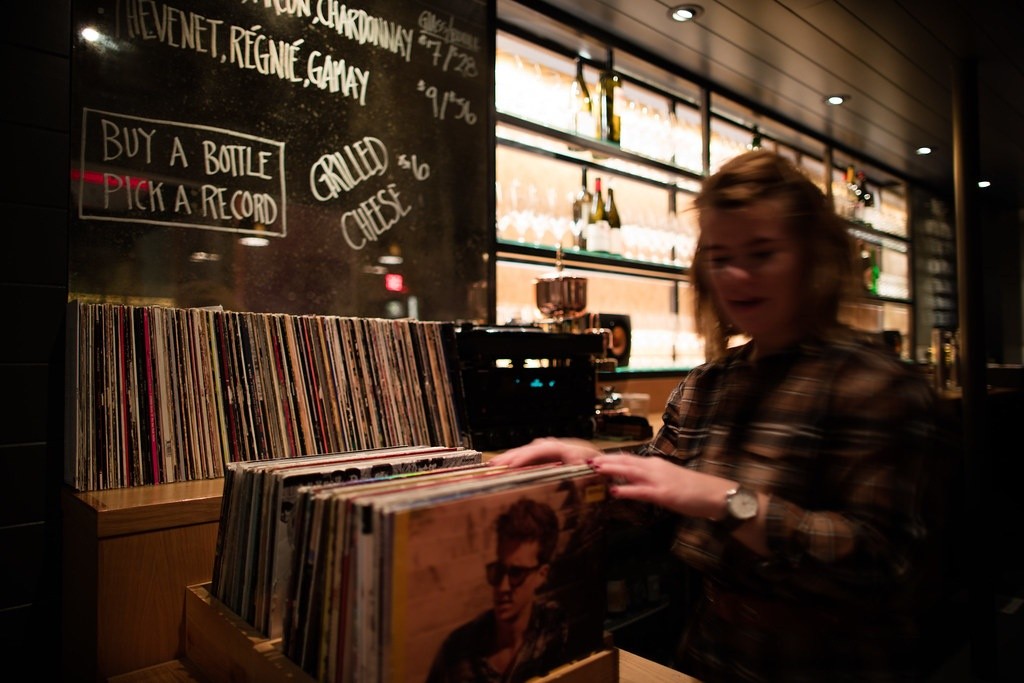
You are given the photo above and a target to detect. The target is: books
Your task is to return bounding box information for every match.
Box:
[68,300,463,491]
[210,446,608,683]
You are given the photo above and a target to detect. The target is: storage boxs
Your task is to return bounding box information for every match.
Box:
[181,578,621,683]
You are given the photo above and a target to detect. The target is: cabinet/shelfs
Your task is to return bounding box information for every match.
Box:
[490,20,918,393]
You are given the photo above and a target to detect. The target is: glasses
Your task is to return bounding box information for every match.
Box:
[486,560,543,588]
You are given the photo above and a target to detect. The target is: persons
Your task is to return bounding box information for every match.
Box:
[493,150,929,683]
[424,498,576,683]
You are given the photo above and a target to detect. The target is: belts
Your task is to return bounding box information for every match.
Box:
[701,579,840,635]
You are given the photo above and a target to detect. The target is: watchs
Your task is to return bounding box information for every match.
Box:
[705,482,758,537]
